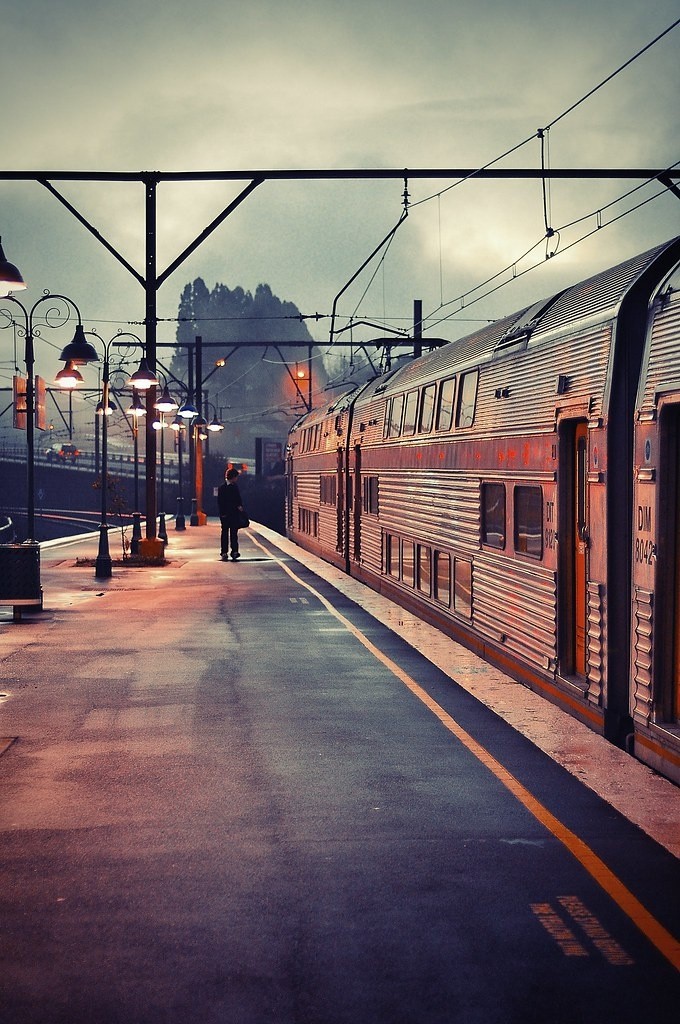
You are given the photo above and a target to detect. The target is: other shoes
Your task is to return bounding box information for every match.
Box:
[231,551,240,558]
[222,553,228,561]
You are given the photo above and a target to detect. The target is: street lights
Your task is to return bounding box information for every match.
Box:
[0,289,101,546]
[95,369,179,552]
[56,328,160,579]
[126,380,224,545]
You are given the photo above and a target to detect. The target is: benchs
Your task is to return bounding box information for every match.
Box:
[0,548,41,620]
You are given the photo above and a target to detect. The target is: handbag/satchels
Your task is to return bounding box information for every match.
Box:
[240,505,249,528]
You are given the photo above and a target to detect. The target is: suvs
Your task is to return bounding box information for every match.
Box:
[46,444,79,464]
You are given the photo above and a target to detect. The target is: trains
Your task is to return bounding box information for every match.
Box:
[281,235,680,786]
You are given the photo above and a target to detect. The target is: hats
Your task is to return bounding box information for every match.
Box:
[224,468,239,479]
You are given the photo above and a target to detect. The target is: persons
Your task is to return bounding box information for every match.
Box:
[217,468,243,559]
